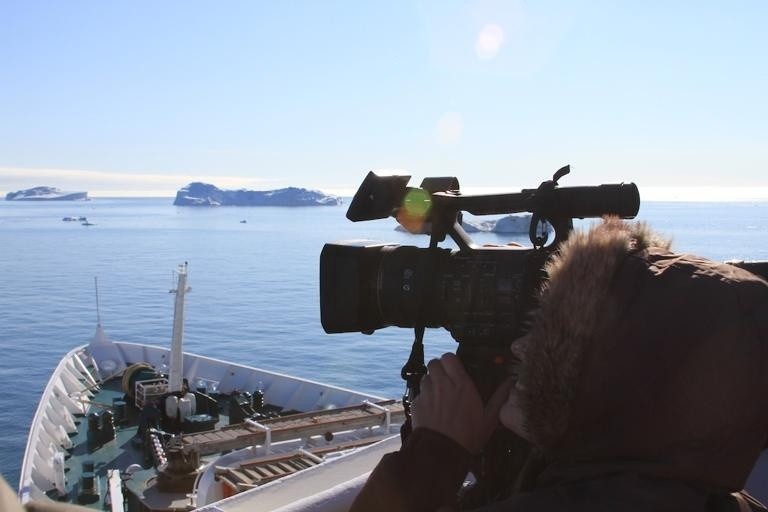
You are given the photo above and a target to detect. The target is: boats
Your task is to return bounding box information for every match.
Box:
[14,259,476,512]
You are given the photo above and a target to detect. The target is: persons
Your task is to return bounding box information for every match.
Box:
[346,216,767,511]
